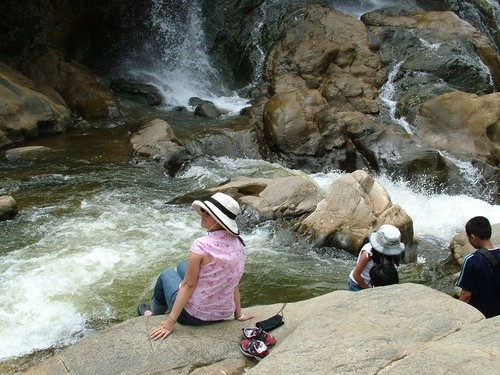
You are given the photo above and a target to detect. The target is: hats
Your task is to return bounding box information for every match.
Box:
[192,192,242,236]
[369,224,405,256]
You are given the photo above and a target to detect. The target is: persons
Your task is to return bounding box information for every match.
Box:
[453,215,500,318]
[348,224,406,291]
[137,190,256,342]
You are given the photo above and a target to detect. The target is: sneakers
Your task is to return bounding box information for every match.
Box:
[239,339,268,360]
[242,326,277,346]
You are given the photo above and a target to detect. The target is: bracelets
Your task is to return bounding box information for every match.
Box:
[234,312,245,320]
[169,314,177,324]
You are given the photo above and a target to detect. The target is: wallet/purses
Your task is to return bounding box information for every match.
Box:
[256,314,284,332]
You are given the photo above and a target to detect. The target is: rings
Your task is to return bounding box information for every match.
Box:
[246,313,249,315]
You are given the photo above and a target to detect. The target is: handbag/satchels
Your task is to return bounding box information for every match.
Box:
[371,264,399,285]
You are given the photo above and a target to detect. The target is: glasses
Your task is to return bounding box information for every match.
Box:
[200,206,211,218]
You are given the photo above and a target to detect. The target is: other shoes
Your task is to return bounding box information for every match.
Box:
[138,304,147,316]
